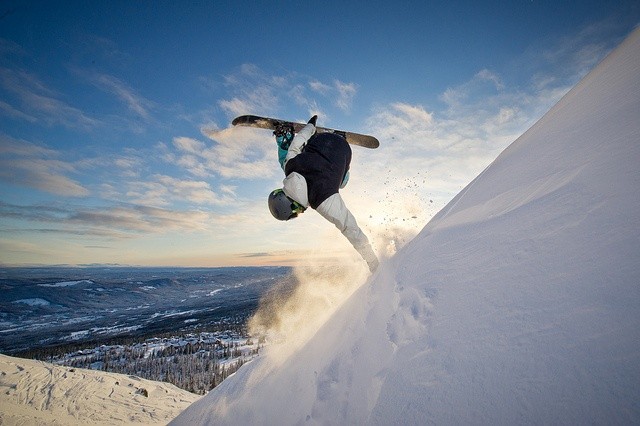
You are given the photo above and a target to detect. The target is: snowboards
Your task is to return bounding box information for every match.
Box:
[232,115,379,148]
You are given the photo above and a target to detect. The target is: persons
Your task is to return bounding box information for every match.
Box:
[268,115,380,273]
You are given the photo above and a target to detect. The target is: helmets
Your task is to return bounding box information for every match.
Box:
[267,188,308,221]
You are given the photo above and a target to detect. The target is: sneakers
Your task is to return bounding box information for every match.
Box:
[333,129,347,138]
[273,119,294,138]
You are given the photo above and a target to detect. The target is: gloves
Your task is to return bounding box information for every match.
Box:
[308,114,318,126]
